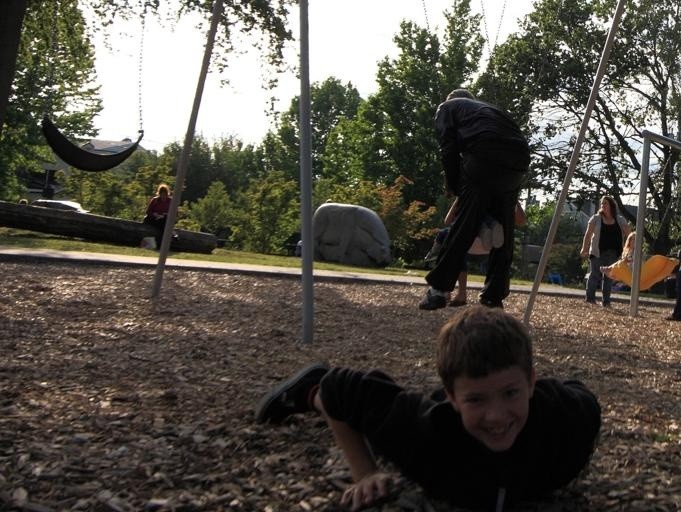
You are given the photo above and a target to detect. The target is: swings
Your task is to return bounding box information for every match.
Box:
[41,1,145,172]
[421,0,499,256]
[610,146,679,291]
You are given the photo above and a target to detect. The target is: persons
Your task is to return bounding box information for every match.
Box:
[665,248,681,321]
[253,304,602,511]
[417,86,527,311]
[440,169,505,251]
[423,195,527,307]
[147,183,183,246]
[599,231,646,279]
[579,196,632,310]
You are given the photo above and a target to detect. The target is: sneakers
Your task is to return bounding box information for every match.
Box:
[253,361,329,426]
[599,266,613,274]
[425,247,439,262]
[481,225,505,250]
[419,290,467,310]
[665,315,681,320]
